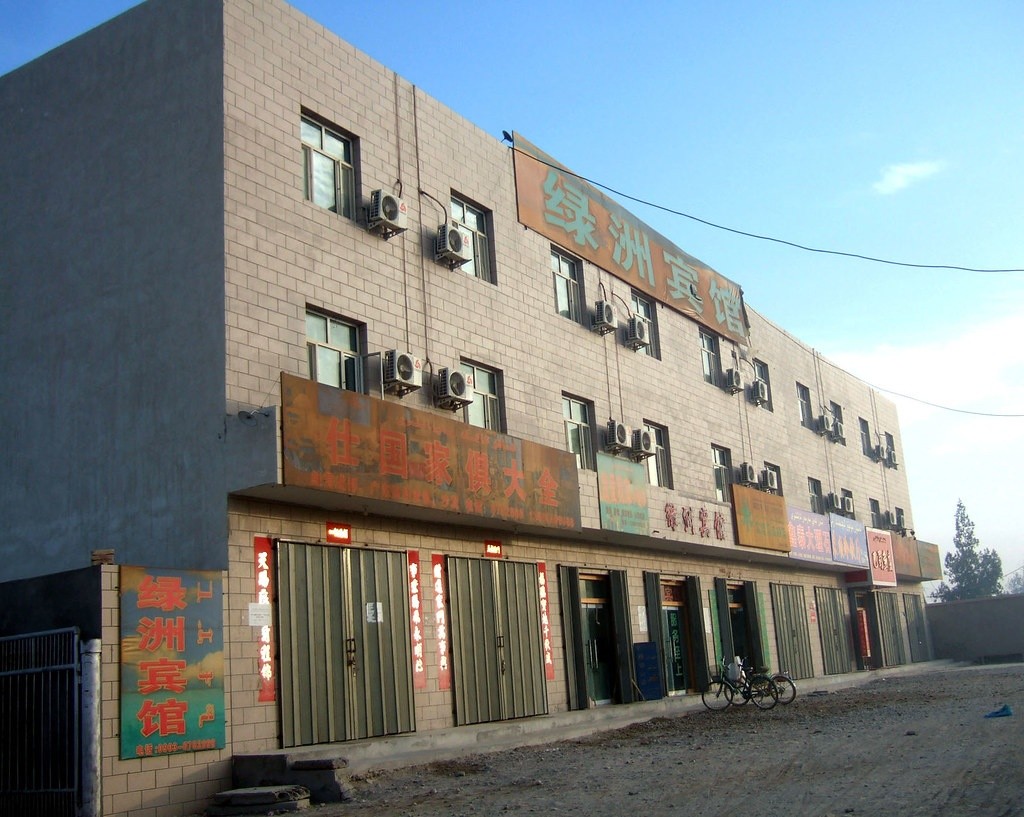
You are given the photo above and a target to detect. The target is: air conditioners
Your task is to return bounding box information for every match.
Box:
[897,515,906,528]
[370,189,408,231]
[827,493,841,510]
[383,349,423,389]
[888,451,899,465]
[752,380,768,404]
[761,469,778,490]
[818,414,832,433]
[739,463,759,484]
[437,367,473,404]
[726,368,745,392]
[875,445,886,459]
[834,422,845,439]
[841,497,853,513]
[886,511,896,525]
[632,429,656,455]
[606,420,633,449]
[436,224,472,263]
[594,300,618,330]
[626,318,650,347]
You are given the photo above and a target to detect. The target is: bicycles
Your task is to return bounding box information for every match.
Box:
[725,655,797,705]
[701,654,778,710]
